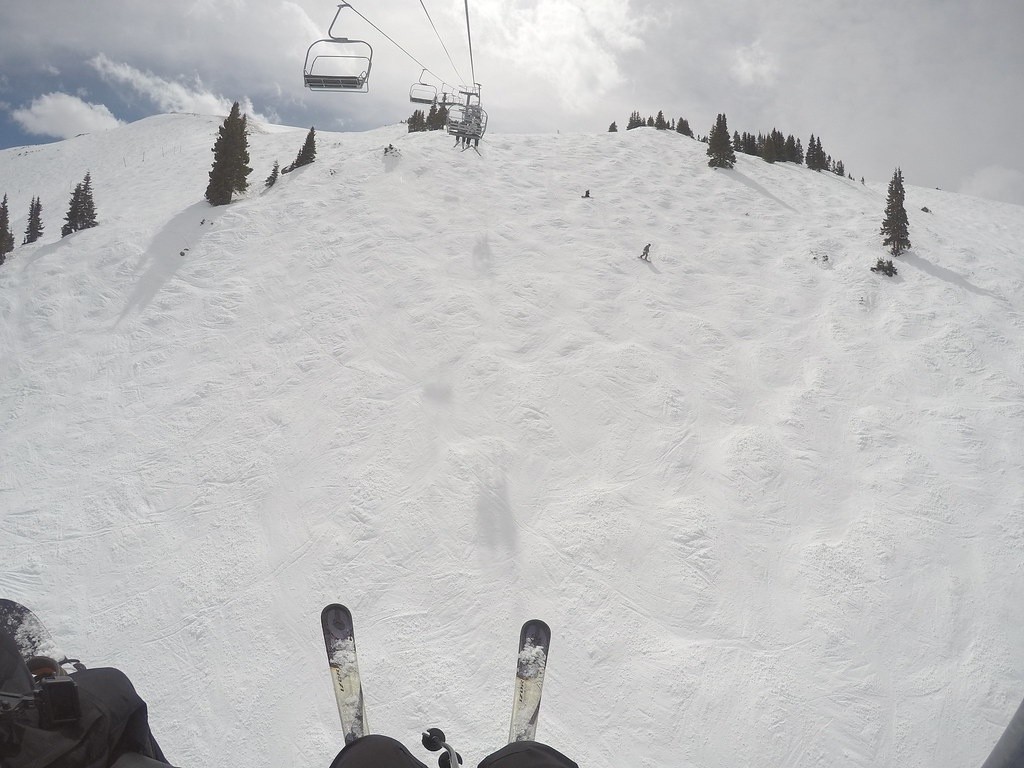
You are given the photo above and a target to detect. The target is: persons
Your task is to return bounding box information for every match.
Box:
[454,115,482,149]
[329,734,579,768]
[0,668,172,768]
[640,244,651,260]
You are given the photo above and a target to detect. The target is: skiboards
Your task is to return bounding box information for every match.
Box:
[321,600,370,745]
[505,614,554,744]
